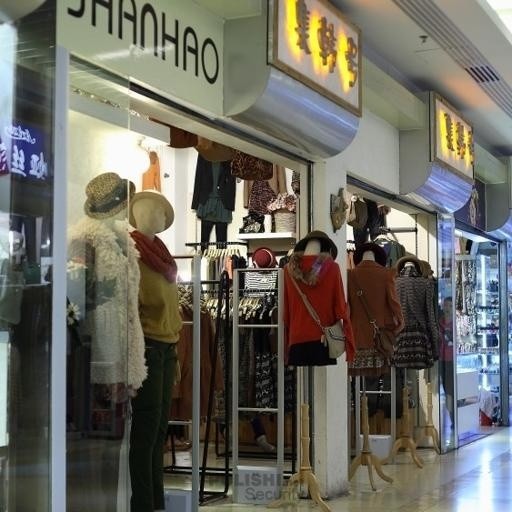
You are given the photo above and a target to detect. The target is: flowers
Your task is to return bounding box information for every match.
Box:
[267,193,297,212]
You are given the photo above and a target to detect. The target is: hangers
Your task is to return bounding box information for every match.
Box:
[200,287,279,321]
[188,241,241,260]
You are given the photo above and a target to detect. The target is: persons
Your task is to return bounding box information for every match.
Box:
[67,174,148,511]
[128,190,182,512]
[348,241,405,376]
[282,231,356,367]
[394,255,440,370]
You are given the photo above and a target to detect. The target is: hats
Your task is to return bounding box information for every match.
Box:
[293,231,338,261]
[83,173,136,220]
[354,242,388,265]
[127,189,174,233]
[395,257,423,275]
[252,247,277,268]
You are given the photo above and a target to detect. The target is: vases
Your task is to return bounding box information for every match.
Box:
[274,211,296,233]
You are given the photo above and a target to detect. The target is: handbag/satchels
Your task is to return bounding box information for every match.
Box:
[285,264,346,359]
[350,270,394,360]
